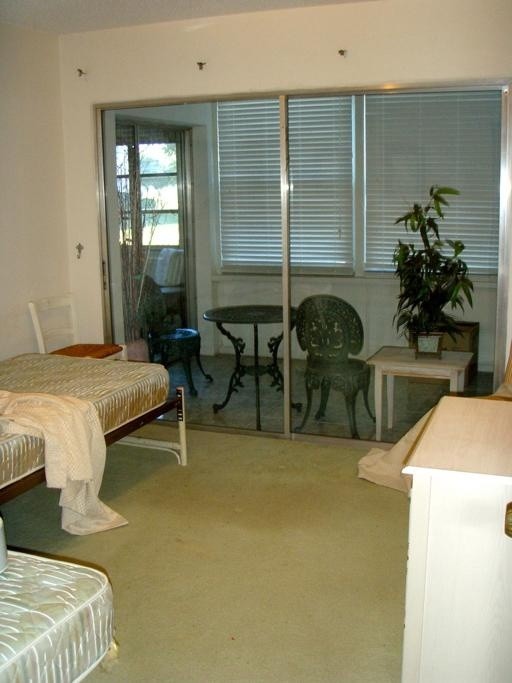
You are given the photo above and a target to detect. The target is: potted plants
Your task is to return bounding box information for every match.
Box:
[391,182,474,359]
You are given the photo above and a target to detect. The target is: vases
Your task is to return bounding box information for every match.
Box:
[127,339,150,362]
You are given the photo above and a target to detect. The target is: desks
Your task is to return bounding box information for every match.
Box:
[203,305,302,415]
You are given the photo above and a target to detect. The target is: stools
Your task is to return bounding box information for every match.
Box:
[159,287,186,329]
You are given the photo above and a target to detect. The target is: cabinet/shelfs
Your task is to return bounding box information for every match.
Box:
[401,396,512,683]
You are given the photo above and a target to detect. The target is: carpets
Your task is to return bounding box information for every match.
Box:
[152,354,493,443]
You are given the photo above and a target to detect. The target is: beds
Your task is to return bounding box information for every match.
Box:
[0,351,188,507]
[0,550,119,683]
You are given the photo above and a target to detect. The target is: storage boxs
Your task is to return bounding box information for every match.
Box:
[410,320,479,386]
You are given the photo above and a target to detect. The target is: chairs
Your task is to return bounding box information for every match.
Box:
[139,276,213,395]
[29,297,127,360]
[294,294,376,439]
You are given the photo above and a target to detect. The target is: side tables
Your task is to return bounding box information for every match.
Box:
[365,346,473,441]
